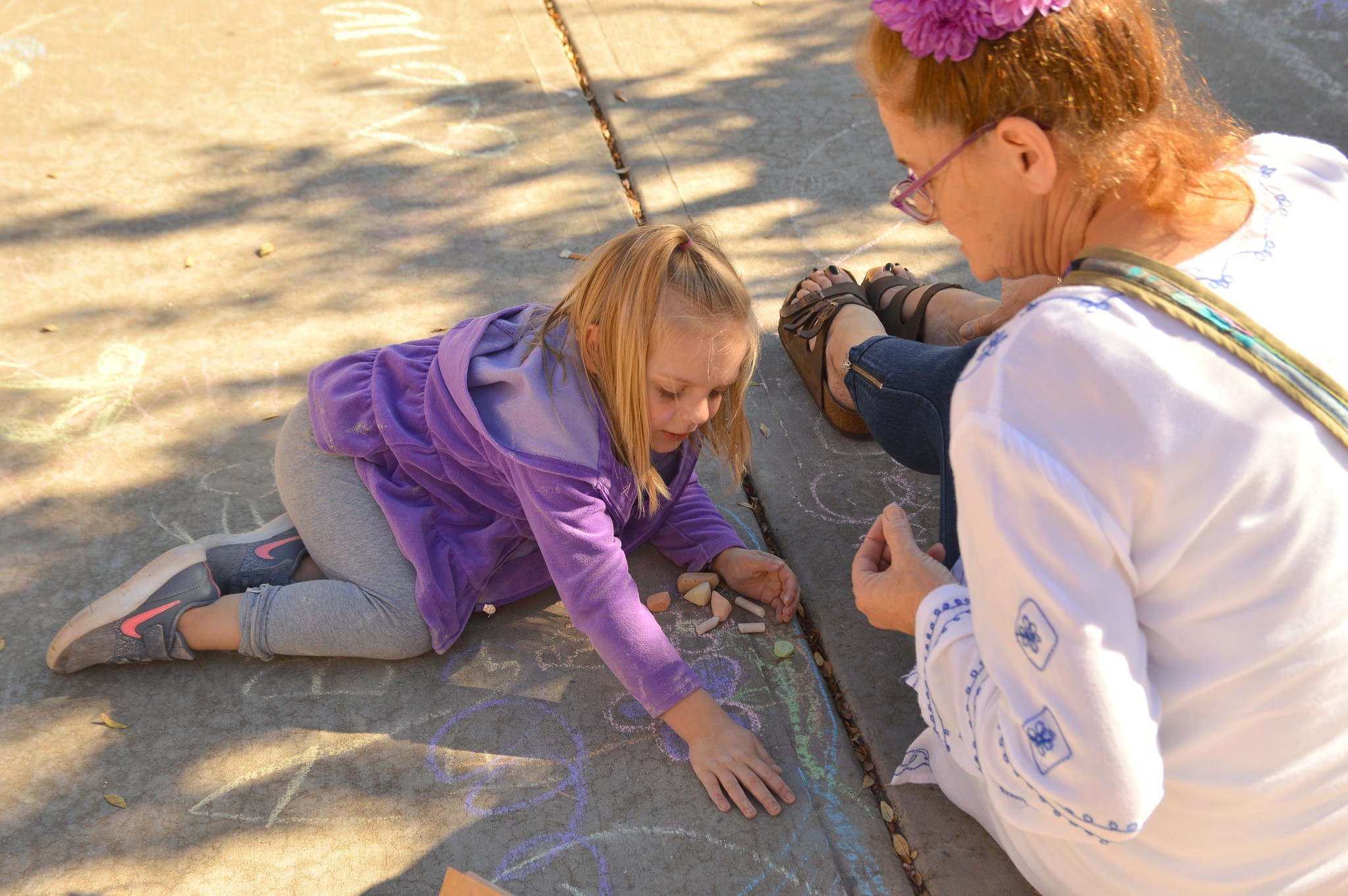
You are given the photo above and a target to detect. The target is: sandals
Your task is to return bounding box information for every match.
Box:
[860,267,973,343]
[778,268,875,441]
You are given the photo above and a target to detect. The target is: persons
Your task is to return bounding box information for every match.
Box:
[46,224,801,815]
[777,0,1348,896]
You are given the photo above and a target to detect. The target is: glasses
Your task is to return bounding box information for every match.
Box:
[888,121,1053,223]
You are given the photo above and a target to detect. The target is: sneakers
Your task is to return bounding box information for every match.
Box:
[194,513,310,593]
[45,544,220,673]
